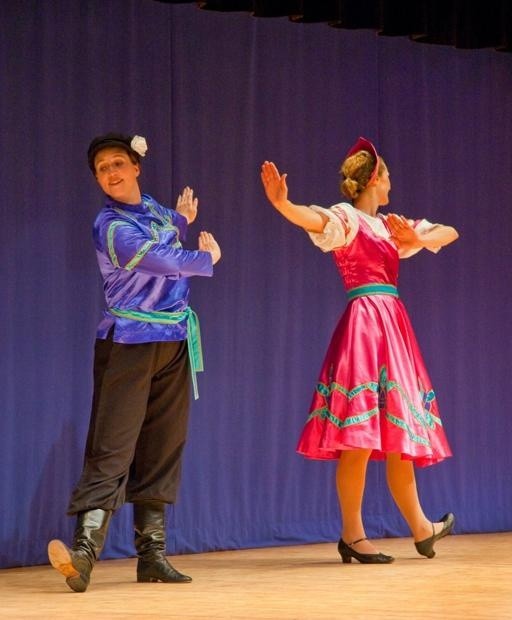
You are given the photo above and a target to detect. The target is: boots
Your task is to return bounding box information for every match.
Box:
[133,499,192,583]
[48,507,113,593]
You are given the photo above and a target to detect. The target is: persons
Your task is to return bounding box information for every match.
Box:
[258,135,460,564]
[46,131,221,594]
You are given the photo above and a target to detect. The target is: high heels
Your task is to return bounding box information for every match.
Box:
[415,512,455,558]
[338,537,395,564]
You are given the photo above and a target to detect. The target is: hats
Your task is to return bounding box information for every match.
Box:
[87,132,147,170]
